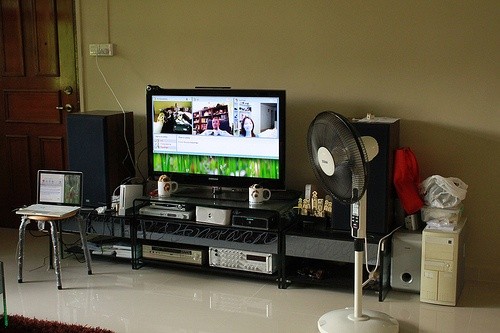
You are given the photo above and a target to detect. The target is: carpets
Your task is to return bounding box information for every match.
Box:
[0,311,113,333]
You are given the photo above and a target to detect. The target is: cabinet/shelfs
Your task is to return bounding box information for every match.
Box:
[81,202,401,303]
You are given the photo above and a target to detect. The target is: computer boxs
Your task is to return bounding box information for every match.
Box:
[420,218,467,305]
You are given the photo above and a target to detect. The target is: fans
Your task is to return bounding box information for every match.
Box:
[306,111,399,333]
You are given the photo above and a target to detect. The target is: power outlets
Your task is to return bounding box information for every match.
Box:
[89,43,113,58]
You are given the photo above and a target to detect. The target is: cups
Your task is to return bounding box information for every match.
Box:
[248,184,271,205]
[158,175,178,198]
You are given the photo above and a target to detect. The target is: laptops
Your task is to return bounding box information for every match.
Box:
[16,170,83,217]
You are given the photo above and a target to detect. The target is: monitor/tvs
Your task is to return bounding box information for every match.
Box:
[147,86,284,201]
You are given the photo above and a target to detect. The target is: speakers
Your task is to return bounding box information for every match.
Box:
[389,228,423,292]
[329,117,400,234]
[67,110,135,209]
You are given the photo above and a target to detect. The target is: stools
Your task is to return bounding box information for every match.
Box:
[18,209,93,290]
